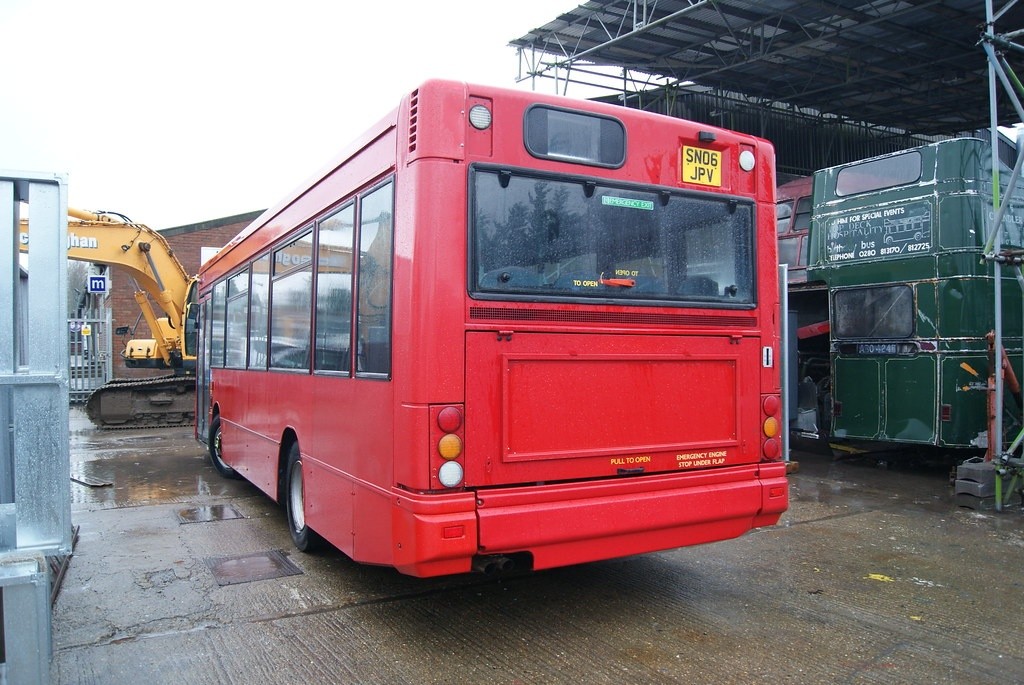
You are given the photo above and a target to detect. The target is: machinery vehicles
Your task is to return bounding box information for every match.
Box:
[19,205,200,430]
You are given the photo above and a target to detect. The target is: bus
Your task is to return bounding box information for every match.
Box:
[193,76,789,579]
[771,136,1024,450]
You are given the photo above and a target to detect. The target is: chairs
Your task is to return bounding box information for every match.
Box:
[674,276,720,298]
[610,276,664,294]
[478,264,541,289]
[550,270,605,292]
